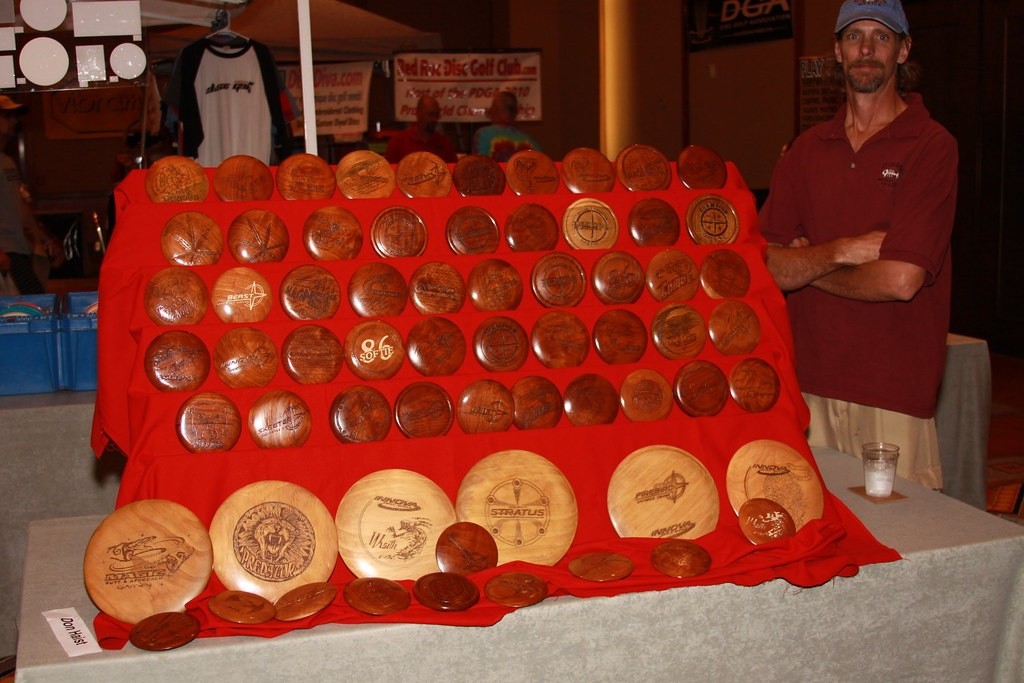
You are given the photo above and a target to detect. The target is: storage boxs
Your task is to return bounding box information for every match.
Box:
[0,291,97,395]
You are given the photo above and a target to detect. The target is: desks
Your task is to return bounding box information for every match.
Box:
[936,332,994,512]
[16,448,1024,683]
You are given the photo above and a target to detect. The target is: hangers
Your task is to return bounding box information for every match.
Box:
[204,8,249,42]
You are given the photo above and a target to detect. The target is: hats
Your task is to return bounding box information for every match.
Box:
[0,95,22,110]
[835,0,909,36]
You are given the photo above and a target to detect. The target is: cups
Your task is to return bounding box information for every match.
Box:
[861,442,900,497]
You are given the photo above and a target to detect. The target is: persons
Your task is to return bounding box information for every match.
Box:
[471,91,552,162]
[0,95,65,295]
[755,1,959,491]
[385,95,459,164]
